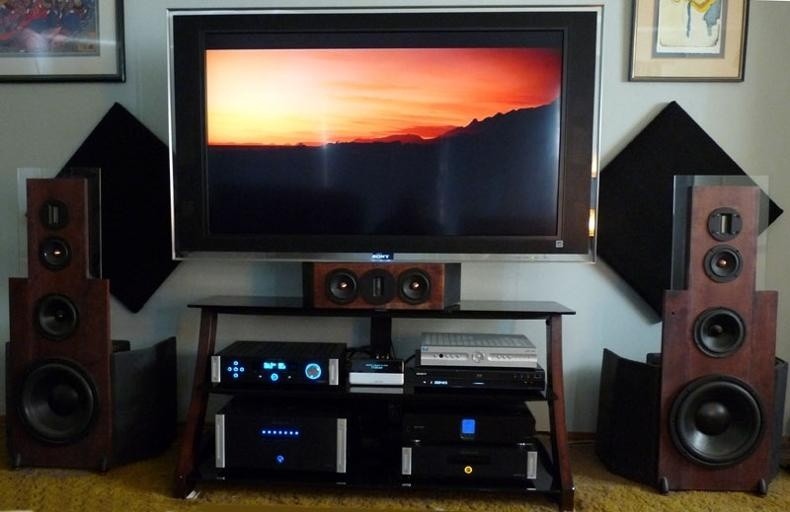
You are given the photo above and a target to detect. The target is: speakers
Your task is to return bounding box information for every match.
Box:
[8,177,109,473]
[314,262,446,310]
[657,185,778,499]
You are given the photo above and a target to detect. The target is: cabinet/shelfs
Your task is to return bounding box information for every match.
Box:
[172,293,576,512]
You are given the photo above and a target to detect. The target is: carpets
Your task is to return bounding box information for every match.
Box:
[0,423,790,512]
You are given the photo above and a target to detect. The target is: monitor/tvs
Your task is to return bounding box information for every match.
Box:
[173,11,597,253]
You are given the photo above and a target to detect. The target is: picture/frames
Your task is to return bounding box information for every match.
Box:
[629,0,750,83]
[0,0,126,83]
